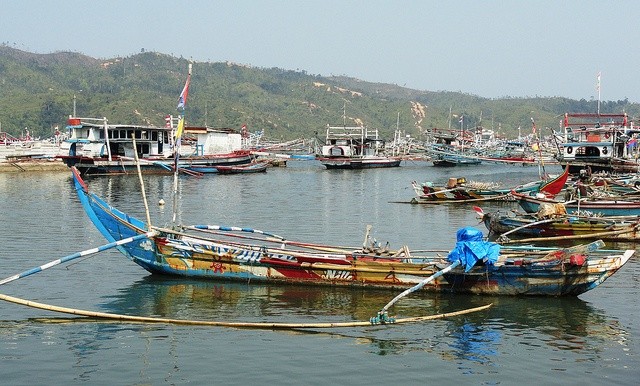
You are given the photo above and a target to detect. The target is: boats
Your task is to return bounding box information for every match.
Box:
[559,77,640,173]
[479,116,563,164]
[472,201,640,243]
[512,161,640,214]
[0,127,67,167]
[0,167,636,327]
[423,104,482,164]
[411,176,516,204]
[55,116,259,175]
[314,103,402,168]
[157,159,272,174]
